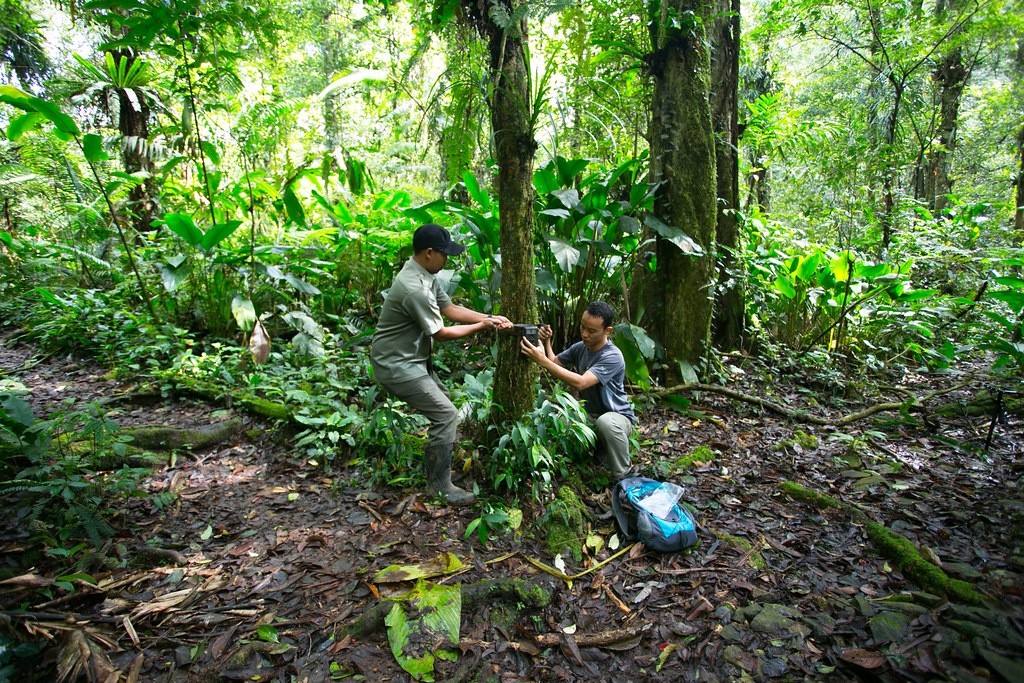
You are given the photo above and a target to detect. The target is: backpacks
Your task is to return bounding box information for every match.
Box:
[612,478,698,552]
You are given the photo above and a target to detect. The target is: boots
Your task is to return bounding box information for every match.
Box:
[424,443,474,506]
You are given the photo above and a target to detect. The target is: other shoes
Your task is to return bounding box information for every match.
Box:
[592,441,607,466]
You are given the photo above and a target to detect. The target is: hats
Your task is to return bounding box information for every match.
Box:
[413,224,464,256]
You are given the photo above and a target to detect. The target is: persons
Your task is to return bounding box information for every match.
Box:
[519,301,637,476]
[370,224,514,508]
